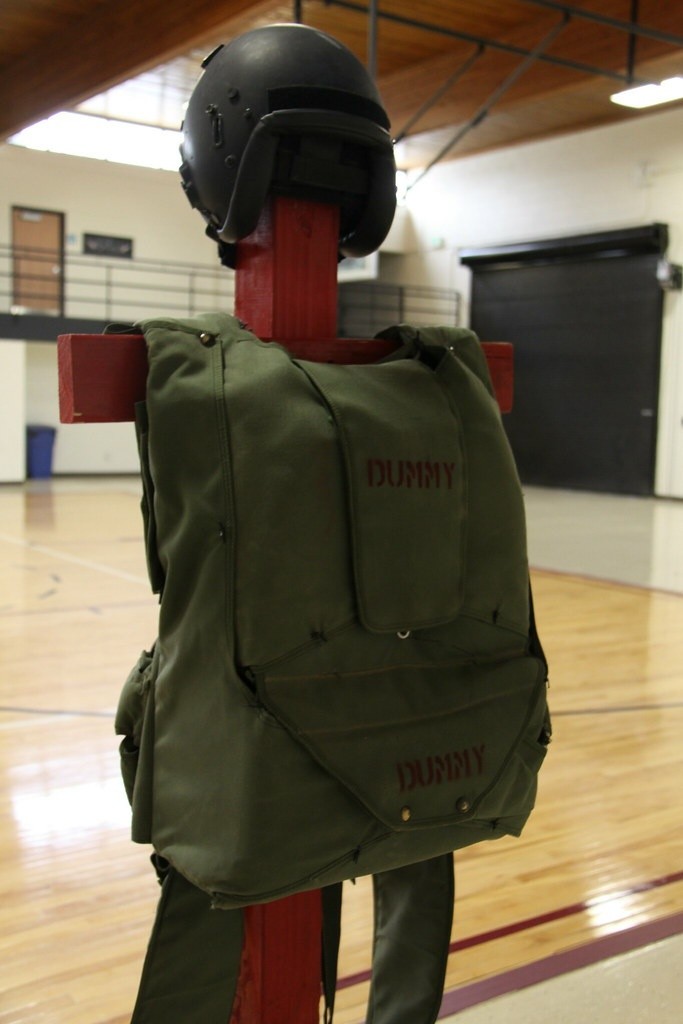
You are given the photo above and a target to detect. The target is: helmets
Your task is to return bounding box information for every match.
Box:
[181,23,395,270]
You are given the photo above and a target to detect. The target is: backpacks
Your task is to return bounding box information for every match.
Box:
[103,308,552,909]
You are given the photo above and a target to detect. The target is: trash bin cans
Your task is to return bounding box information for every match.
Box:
[26,425,56,479]
